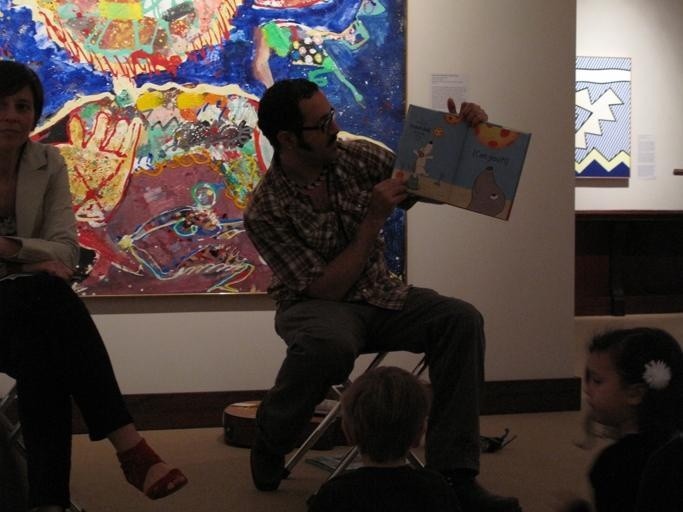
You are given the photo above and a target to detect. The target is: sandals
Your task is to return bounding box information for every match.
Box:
[117,439,187,500]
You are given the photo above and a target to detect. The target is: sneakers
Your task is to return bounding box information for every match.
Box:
[251,430,282,491]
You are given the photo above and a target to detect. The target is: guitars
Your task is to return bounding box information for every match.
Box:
[223,399,516,452]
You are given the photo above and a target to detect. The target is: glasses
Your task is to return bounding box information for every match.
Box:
[296,107,334,131]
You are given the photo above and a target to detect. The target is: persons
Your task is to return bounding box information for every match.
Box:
[0,62,188,512]
[551,327,683,512]
[244,79,523,512]
[306,366,464,512]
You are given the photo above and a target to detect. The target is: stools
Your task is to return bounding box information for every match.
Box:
[282,351,428,504]
[0,372,88,512]
